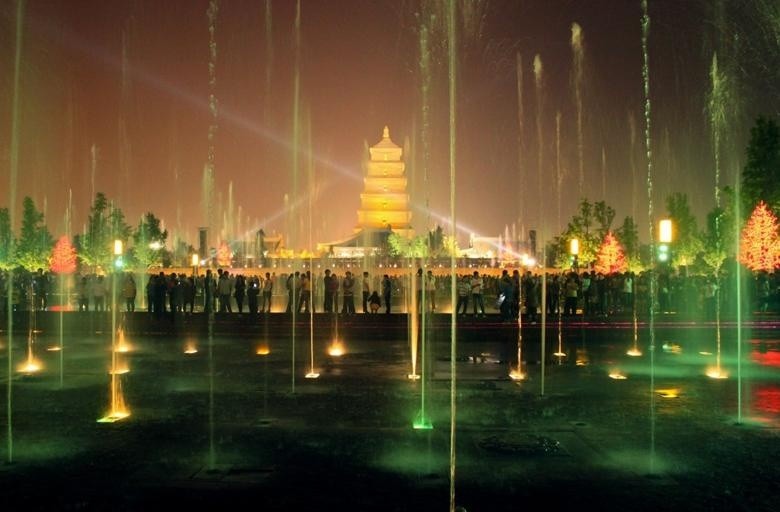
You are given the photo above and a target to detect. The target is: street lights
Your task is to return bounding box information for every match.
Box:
[658,218,674,274]
[570,237,580,272]
[192,254,199,277]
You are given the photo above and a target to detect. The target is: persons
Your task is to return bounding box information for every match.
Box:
[322,269,437,313]
[77,274,136,312]
[145,267,316,313]
[0,426,780,512]
[456,265,780,317]
[0,264,51,310]
